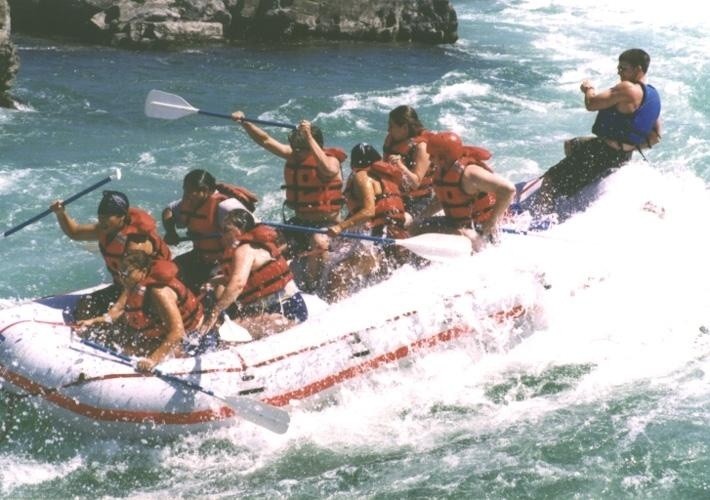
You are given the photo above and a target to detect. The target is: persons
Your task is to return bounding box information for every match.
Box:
[410,131,517,270]
[538,49,662,214]
[161,168,258,298]
[293,142,411,302]
[52,189,172,320]
[232,109,347,259]
[200,208,308,348]
[382,106,437,217]
[72,250,219,376]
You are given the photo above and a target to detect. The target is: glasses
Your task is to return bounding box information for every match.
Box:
[116,268,138,280]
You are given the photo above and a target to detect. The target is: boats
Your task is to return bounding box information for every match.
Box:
[1,155,679,436]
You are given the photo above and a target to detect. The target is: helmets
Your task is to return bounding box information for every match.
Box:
[351,142,382,169]
[97,190,129,216]
[426,133,464,162]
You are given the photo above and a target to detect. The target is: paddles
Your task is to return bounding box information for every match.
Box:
[71,330,291,433]
[207,287,253,342]
[259,221,472,264]
[145,89,301,132]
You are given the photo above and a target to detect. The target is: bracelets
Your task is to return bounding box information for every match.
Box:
[585,85,595,90]
[214,304,222,311]
[482,233,489,240]
[348,217,354,225]
